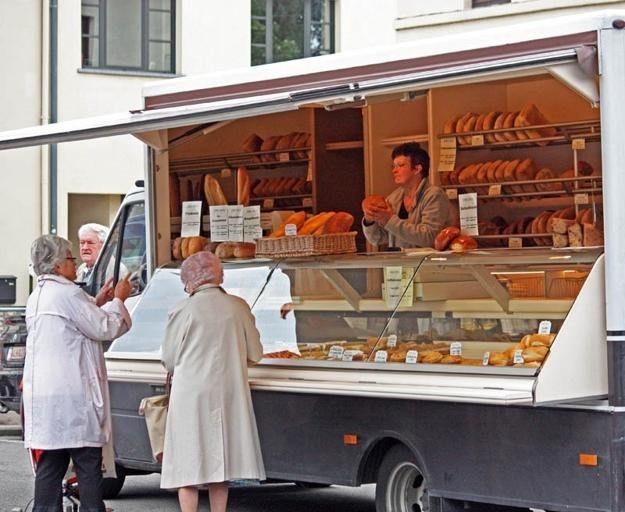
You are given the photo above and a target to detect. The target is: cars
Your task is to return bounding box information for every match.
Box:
[0,307,26,412]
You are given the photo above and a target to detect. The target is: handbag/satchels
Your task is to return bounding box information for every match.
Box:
[138,371,171,463]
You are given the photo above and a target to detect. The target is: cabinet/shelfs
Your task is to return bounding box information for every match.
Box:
[103,116,609,408]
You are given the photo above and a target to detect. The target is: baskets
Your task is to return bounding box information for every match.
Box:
[506,270,588,298]
[253,231,359,258]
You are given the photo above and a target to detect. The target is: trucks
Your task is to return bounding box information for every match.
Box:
[0,10,625,512]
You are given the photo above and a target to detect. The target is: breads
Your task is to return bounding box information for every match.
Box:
[166,102,605,365]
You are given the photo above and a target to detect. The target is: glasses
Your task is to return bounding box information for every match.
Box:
[63,257,75,264]
[184,280,190,293]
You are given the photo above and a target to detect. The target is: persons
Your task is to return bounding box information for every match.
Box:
[21,233,134,512]
[360,141,451,248]
[76,222,131,297]
[158,250,268,512]
